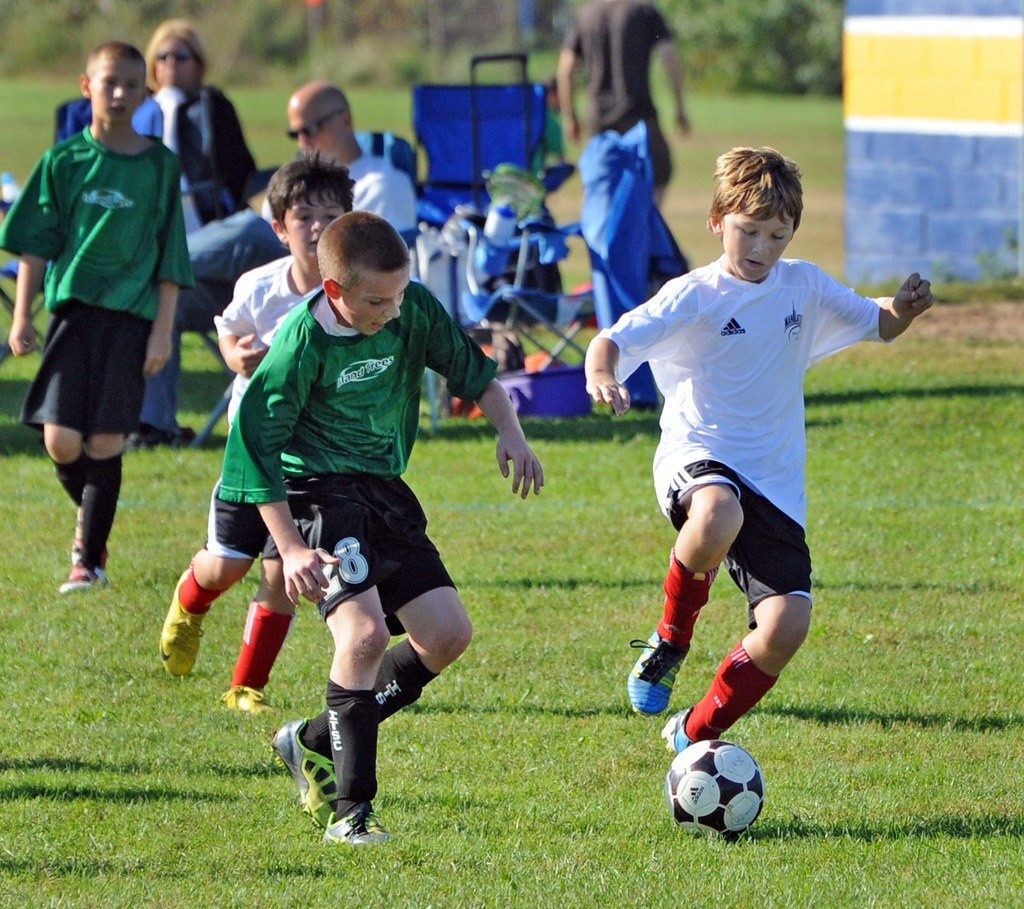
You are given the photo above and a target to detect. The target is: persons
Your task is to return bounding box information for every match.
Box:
[218,211,544,845]
[556,0,691,209]
[124,81,418,448]
[0,43,197,601]
[161,162,354,714]
[149,19,255,221]
[584,145,933,756]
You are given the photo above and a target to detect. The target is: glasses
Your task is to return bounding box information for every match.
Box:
[287,106,345,140]
[153,48,196,64]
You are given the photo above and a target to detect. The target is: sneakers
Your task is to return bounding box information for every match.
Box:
[220,680,281,718]
[624,631,692,719]
[659,702,696,759]
[320,808,392,849]
[269,718,340,836]
[158,569,204,679]
[61,549,107,596]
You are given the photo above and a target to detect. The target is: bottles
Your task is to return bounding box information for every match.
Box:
[484,203,519,244]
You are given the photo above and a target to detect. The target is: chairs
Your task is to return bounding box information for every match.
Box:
[2,83,688,445]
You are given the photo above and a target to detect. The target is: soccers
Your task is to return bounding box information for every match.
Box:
[663,740,769,837]
